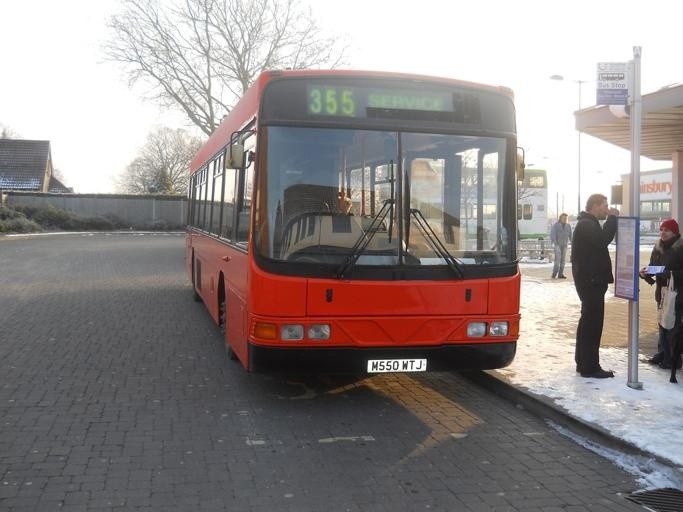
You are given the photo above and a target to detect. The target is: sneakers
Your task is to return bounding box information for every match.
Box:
[552,274,567,278]
[650,357,667,369]
[577,363,613,377]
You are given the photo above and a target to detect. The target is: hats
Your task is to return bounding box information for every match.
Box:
[659,219,679,235]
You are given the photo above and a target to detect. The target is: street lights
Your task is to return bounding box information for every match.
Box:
[548,73,589,222]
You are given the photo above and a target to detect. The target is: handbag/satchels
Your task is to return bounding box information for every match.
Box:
[656,286,678,329]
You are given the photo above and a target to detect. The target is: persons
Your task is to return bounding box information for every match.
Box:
[638,219,683,368]
[551,213,572,278]
[572,194,619,378]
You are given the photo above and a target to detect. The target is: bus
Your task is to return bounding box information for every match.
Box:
[181,65,530,380]
[375,161,551,244]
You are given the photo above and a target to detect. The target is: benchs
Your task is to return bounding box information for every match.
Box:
[272,198,388,258]
